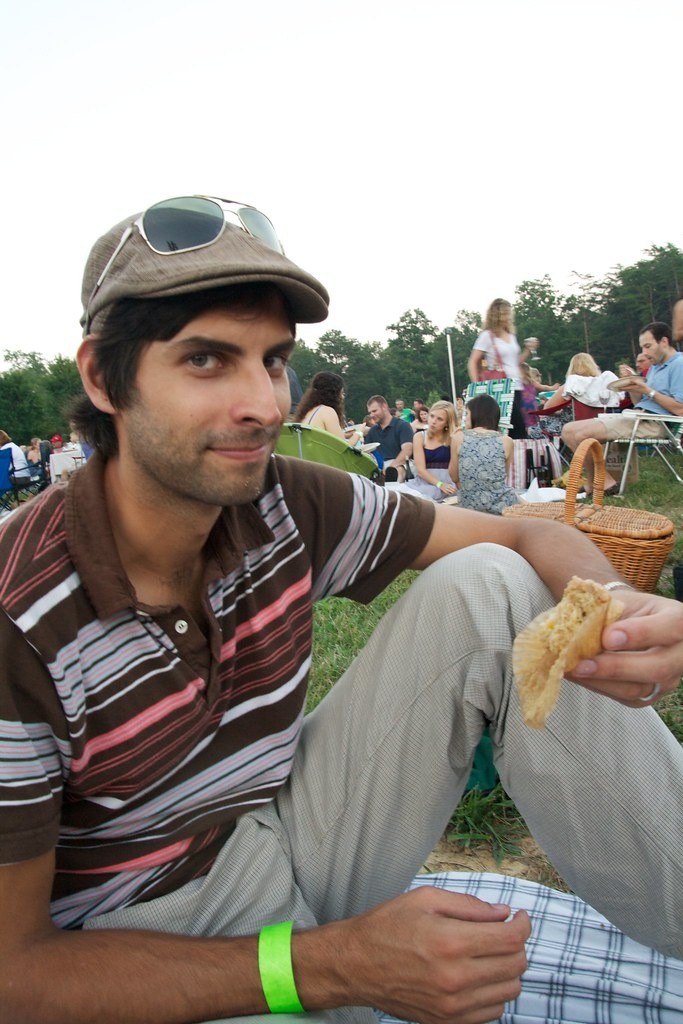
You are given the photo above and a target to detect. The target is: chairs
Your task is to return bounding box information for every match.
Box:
[0,439,54,516]
[526,369,614,478]
[599,413,683,498]
[274,423,380,484]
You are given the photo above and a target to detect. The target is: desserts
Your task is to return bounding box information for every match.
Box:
[511,574,623,729]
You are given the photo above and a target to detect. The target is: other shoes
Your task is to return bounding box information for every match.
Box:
[586,482,619,498]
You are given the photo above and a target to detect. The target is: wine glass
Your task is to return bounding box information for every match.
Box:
[524,337,541,360]
[599,390,610,414]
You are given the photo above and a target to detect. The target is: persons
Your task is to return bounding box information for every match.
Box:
[0,207,683,1024]
[361,395,414,483]
[448,394,524,515]
[396,399,416,422]
[348,398,423,427]
[530,367,542,383]
[286,366,368,447]
[412,406,429,433]
[0,419,80,489]
[519,361,563,438]
[625,353,652,399]
[413,400,459,495]
[543,352,601,410]
[562,322,683,497]
[467,298,527,439]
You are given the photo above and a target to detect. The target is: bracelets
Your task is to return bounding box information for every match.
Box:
[605,581,635,590]
[649,389,655,398]
[355,431,363,437]
[436,482,442,487]
[259,920,305,1012]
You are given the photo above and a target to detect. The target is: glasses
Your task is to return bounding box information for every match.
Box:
[85,195,286,340]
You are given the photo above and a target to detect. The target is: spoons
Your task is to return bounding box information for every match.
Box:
[624,366,639,376]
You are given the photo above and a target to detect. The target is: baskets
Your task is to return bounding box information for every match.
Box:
[500,438,677,593]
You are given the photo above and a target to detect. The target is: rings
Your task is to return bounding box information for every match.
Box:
[641,684,661,701]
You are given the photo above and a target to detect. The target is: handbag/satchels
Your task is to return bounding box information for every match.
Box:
[478,370,507,381]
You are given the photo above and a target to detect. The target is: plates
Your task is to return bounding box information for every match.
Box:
[359,442,380,453]
[607,377,648,392]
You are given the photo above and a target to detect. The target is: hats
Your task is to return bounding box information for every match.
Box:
[51,435,62,443]
[80,210,330,338]
